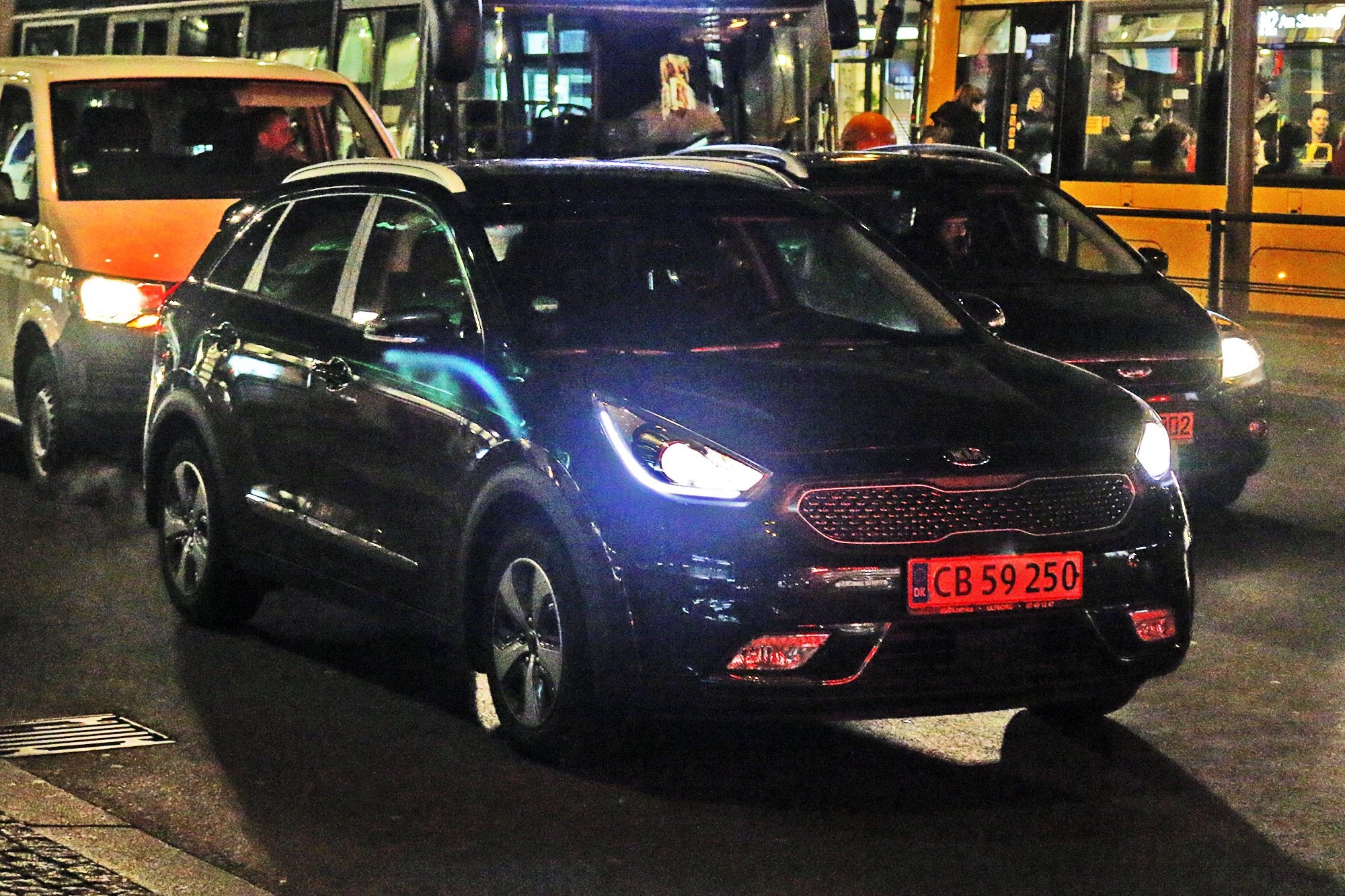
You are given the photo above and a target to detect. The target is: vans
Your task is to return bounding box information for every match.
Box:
[0,53,421,477]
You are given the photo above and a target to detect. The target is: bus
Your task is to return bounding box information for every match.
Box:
[917,1,1344,319]
[5,0,910,145]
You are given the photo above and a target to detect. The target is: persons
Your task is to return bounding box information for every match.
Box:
[932,215,974,256]
[1087,64,1198,174]
[918,82,985,148]
[840,112,898,156]
[225,108,312,193]
[1251,77,1345,189]
[1010,34,1062,171]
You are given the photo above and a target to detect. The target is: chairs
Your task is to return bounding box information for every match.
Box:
[64,106,161,198]
[387,228,511,352]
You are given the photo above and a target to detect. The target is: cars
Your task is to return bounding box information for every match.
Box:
[136,145,1194,754]
[674,135,1271,525]
